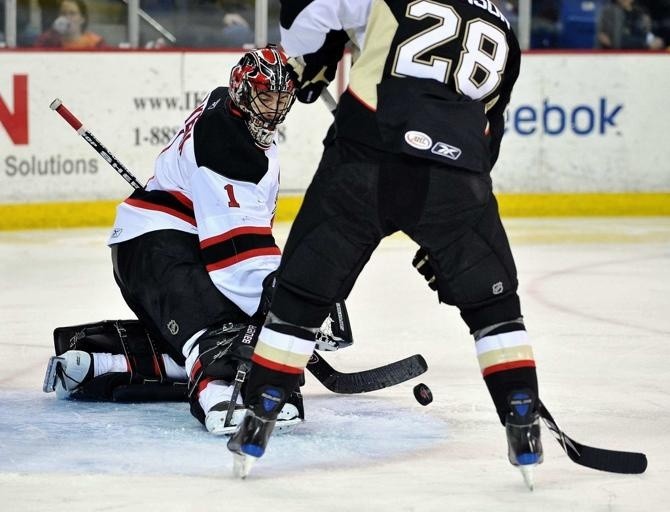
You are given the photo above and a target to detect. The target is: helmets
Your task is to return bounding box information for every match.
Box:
[227,47,298,150]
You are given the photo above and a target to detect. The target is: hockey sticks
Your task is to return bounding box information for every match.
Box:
[319,90,647,475]
[51,98,429,395]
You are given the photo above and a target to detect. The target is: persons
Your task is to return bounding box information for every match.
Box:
[54,46,355,437]
[226,0,544,468]
[31,0,107,48]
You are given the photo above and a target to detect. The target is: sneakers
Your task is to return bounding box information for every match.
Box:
[504,387,546,468]
[204,383,302,460]
[54,349,115,402]
